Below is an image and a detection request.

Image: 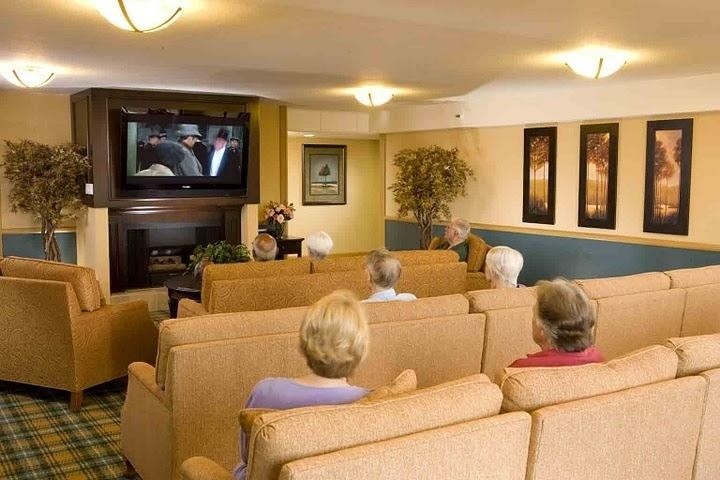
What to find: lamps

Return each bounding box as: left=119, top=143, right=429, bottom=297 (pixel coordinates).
left=87, top=0, right=196, bottom=39
left=556, top=43, right=633, bottom=81
left=350, top=84, right=398, bottom=110
left=0, top=57, right=62, bottom=94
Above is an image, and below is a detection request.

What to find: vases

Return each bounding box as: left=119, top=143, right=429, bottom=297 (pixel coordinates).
left=264, top=223, right=286, bottom=239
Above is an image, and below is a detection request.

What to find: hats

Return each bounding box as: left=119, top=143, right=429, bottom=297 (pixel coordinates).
left=148, top=131, right=161, bottom=138
left=216, top=128, right=230, bottom=141
left=149, top=142, right=185, bottom=166
left=174, top=124, right=202, bottom=137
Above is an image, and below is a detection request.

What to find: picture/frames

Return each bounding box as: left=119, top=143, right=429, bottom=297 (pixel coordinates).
left=521, top=122, right=558, bottom=227
left=299, top=141, right=349, bottom=209
left=574, top=123, right=620, bottom=229
left=642, top=114, right=695, bottom=237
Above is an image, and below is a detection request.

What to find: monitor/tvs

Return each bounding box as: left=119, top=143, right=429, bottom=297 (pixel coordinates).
left=120, top=113, right=249, bottom=189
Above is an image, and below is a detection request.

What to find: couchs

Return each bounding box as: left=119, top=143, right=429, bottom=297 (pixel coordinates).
left=665, top=330, right=719, bottom=478
left=116, top=292, right=490, bottom=477
left=661, top=263, right=719, bottom=336
left=171, top=244, right=472, bottom=315
left=175, top=365, right=532, bottom=480
left=0, top=246, right=164, bottom=413
left=461, top=269, right=689, bottom=379
left=488, top=343, right=711, bottom=480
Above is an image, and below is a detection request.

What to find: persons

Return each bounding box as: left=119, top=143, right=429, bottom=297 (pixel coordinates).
left=485, top=246, right=529, bottom=289
left=356, top=248, right=417, bottom=303
left=251, top=235, right=279, bottom=260
left=436, top=218, right=471, bottom=262
left=234, top=288, right=372, bottom=478
left=306, top=229, right=333, bottom=257
left=135, top=125, right=243, bottom=177
left=509, top=275, right=607, bottom=367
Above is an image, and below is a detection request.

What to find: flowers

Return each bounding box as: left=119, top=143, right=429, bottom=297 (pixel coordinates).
left=261, top=197, right=300, bottom=225
